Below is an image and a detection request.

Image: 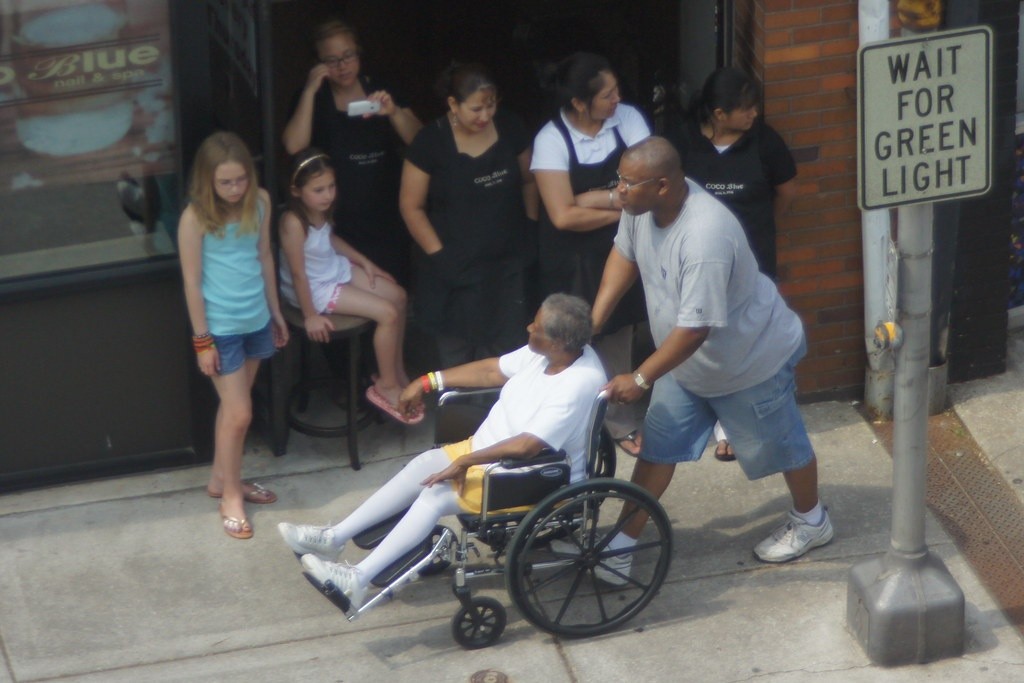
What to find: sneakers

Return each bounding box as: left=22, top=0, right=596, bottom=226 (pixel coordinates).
left=278, top=522, right=345, bottom=563
left=301, top=553, right=367, bottom=611
left=549, top=532, right=632, bottom=587
left=753, top=503, right=833, bottom=564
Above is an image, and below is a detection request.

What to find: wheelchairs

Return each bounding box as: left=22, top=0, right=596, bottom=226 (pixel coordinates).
left=294, top=384, right=674, bottom=652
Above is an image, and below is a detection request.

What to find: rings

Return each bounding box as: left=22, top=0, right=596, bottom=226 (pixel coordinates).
left=617, top=400, right=624, bottom=405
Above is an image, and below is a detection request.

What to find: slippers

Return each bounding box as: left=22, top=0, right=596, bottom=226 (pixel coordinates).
left=219, top=502, right=253, bottom=539
left=366, top=386, right=424, bottom=424
left=207, top=483, right=277, bottom=503
left=371, top=373, right=426, bottom=412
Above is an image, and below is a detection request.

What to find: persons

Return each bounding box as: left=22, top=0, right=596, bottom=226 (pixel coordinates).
left=665, top=68, right=799, bottom=460
left=524, top=50, right=652, bottom=457
left=276, top=147, right=426, bottom=426
left=278, top=291, right=608, bottom=609
left=588, top=137, right=835, bottom=587
left=396, top=67, right=538, bottom=391
left=176, top=131, right=290, bottom=540
left=282, top=20, right=439, bottom=371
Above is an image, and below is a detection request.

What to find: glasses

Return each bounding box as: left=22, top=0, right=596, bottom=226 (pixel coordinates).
left=316, top=47, right=360, bottom=68
left=616, top=170, right=661, bottom=193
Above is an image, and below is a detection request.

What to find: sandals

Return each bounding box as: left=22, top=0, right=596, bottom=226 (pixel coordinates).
left=613, top=429, right=642, bottom=456
left=715, top=438, right=735, bottom=461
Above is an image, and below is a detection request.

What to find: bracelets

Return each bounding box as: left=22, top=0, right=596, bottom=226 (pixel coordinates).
left=427, top=372, right=437, bottom=390
left=633, top=369, right=650, bottom=390
left=420, top=375, right=431, bottom=394
left=191, top=331, right=216, bottom=355
left=435, top=370, right=443, bottom=391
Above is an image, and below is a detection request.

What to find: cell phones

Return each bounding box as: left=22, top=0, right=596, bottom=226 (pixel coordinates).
left=348, top=99, right=380, bottom=116
